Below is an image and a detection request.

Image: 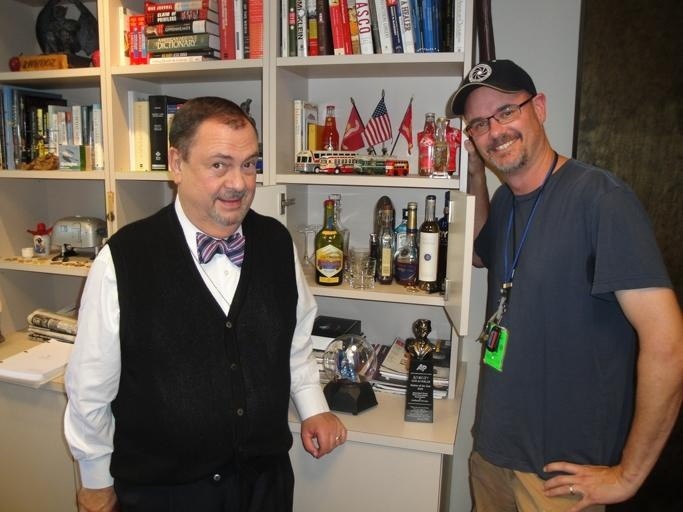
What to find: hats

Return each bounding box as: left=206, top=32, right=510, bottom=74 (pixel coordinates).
left=451, top=59, right=536, bottom=115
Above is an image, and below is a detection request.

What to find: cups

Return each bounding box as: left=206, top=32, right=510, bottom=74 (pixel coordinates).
left=22, top=247, right=33, bottom=259
left=345, top=247, right=376, bottom=290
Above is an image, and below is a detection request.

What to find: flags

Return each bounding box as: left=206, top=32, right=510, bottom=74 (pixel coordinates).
left=398, top=103, right=413, bottom=155
left=341, top=106, right=365, bottom=152
left=363, top=96, right=394, bottom=148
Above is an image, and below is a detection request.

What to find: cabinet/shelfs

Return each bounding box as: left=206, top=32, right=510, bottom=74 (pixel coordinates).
left=0, top=0, right=472, bottom=512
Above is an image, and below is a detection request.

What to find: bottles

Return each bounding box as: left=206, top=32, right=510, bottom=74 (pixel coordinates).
left=436, top=191, right=449, bottom=292
left=416, top=194, right=441, bottom=294
left=394, top=201, right=420, bottom=288
left=313, top=193, right=351, bottom=287
left=321, top=105, right=339, bottom=150
left=367, top=233, right=376, bottom=278
left=393, top=208, right=407, bottom=235
left=375, top=203, right=397, bottom=285
left=32, top=222, right=51, bottom=257
left=417, top=112, right=461, bottom=178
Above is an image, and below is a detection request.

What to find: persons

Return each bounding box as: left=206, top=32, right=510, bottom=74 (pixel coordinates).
left=450, top=56, right=683, bottom=512
left=63, top=95, right=348, bottom=512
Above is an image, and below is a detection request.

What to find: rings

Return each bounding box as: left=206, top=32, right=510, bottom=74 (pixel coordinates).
left=569, top=484, right=575, bottom=495
left=336, top=435, right=343, bottom=441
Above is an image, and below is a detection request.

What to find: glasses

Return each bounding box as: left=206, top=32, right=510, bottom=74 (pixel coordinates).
left=466, top=97, right=533, bottom=137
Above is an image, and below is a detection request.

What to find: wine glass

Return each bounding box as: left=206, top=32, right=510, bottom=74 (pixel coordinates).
left=296, top=222, right=314, bottom=280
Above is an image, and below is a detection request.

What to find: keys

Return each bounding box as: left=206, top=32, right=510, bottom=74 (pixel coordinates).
left=475, top=320, right=501, bottom=353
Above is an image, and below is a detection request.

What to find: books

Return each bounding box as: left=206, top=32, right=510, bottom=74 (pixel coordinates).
left=310, top=314, right=451, bottom=400
left=8, top=51, right=91, bottom=72
left=0, top=85, right=189, bottom=172
left=125, top=2, right=264, bottom=66
left=293, top=99, right=320, bottom=172
left=279, top=2, right=455, bottom=57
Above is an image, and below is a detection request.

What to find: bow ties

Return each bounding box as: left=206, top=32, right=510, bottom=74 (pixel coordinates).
left=196, top=232, right=245, bottom=267
